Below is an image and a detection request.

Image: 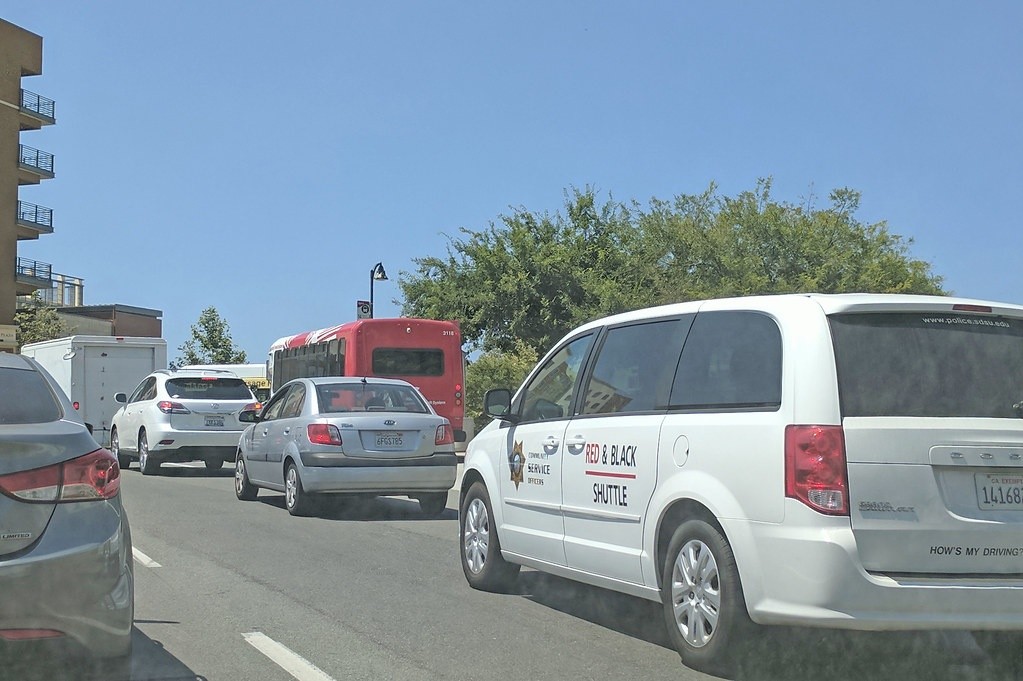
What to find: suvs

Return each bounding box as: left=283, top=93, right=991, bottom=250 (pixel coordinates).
left=109, top=368, right=266, bottom=477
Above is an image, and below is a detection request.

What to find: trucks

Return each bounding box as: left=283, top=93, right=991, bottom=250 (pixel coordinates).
left=20, top=335, right=168, bottom=450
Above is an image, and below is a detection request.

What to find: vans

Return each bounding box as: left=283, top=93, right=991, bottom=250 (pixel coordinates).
left=458, top=293, right=1023, bottom=674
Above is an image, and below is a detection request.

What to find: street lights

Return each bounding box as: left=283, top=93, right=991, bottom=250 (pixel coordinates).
left=371, top=261, right=388, bottom=320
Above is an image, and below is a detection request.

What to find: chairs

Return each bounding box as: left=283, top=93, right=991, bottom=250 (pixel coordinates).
left=364, top=398, right=385, bottom=410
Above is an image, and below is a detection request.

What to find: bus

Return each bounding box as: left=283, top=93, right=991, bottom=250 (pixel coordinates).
left=266, top=317, right=464, bottom=432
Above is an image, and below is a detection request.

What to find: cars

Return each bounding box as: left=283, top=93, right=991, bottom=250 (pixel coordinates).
left=0, top=353, right=134, bottom=681
left=233, top=376, right=458, bottom=518
